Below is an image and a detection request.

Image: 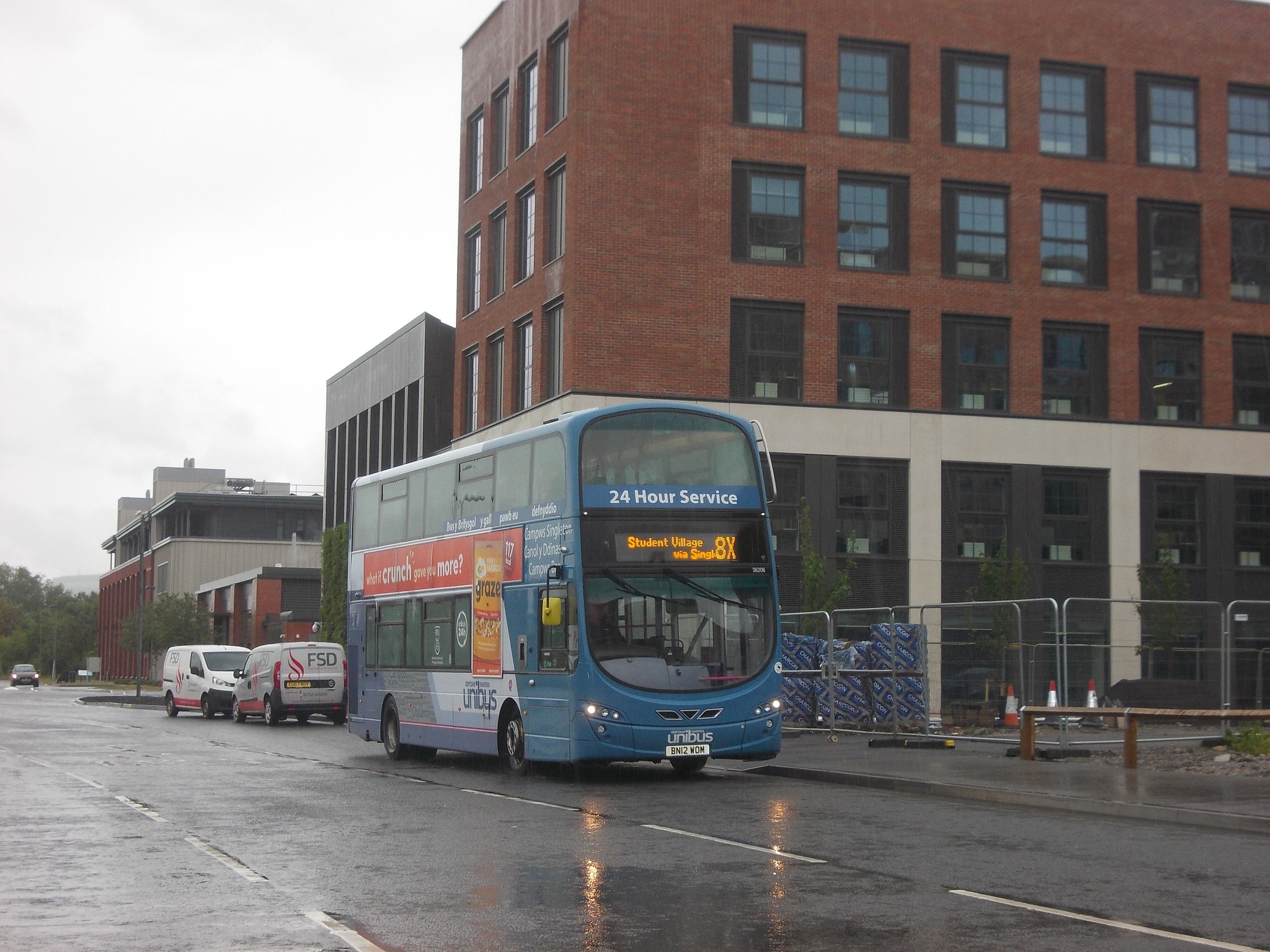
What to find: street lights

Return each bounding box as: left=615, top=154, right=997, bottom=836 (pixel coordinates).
left=49, top=605, right=57, bottom=678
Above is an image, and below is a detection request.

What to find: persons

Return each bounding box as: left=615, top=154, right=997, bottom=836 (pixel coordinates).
left=586, top=602, right=627, bottom=652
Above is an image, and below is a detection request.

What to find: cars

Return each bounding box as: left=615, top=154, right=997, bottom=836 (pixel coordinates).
left=10, top=664, right=41, bottom=687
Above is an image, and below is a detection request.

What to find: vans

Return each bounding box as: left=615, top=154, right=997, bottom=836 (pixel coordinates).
left=162, top=645, right=252, bottom=719
left=231, top=641, right=348, bottom=727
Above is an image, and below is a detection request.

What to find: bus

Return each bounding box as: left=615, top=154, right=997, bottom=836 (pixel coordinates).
left=347, top=402, right=784, bottom=778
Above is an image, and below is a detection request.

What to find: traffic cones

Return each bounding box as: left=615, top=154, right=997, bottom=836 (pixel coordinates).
left=1036, top=680, right=1068, bottom=726
left=1075, top=679, right=1105, bottom=727
left=997, top=684, right=1020, bottom=730
